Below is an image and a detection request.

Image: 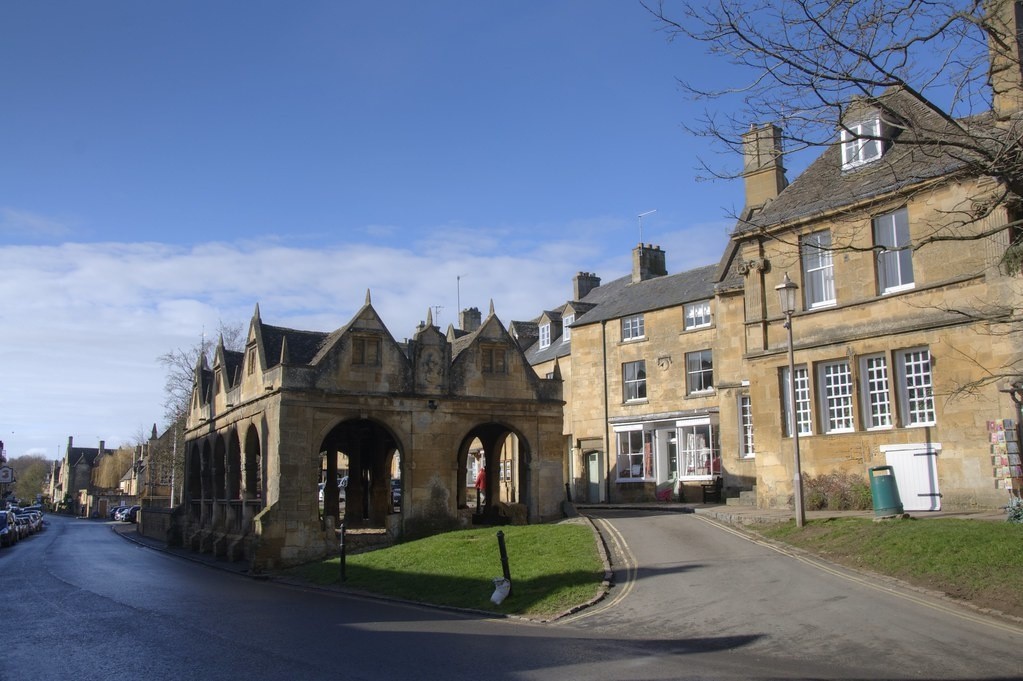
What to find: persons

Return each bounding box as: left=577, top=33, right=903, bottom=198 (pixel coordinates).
left=7, top=508, right=15, bottom=526
left=475, top=467, right=486, bottom=505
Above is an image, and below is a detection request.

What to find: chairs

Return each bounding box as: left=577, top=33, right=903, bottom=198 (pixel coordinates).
left=701, top=476, right=720, bottom=503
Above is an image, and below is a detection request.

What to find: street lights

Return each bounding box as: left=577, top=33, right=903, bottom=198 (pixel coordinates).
left=775, top=272, right=805, bottom=527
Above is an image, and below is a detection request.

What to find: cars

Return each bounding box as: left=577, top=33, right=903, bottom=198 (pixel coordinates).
left=110, top=505, right=141, bottom=524
left=0, top=505, right=44, bottom=548
left=390, top=480, right=402, bottom=506
left=317, top=476, right=349, bottom=509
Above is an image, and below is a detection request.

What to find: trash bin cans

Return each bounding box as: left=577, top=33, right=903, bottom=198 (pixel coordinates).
left=868, top=465, right=904, bottom=520
